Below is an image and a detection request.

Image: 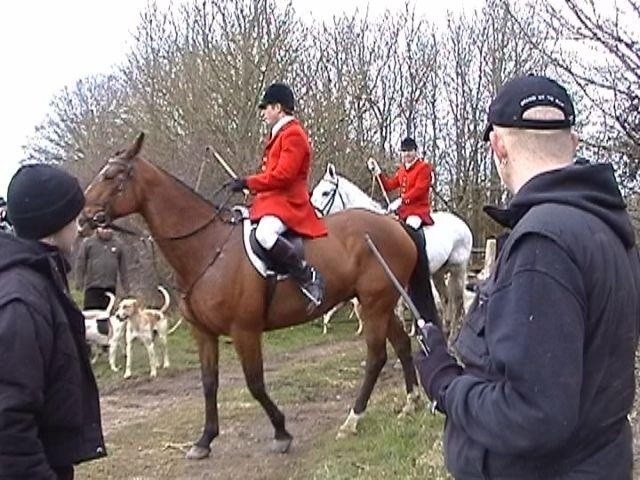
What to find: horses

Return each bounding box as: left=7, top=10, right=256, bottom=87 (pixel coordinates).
left=74, top=130, right=443, bottom=461
left=310, top=162, right=474, bottom=370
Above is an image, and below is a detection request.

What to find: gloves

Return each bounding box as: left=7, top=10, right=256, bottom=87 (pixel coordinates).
left=389, top=197, right=402, bottom=211
left=367, top=157, right=381, bottom=177
left=230, top=178, right=246, bottom=192
left=407, top=325, right=466, bottom=414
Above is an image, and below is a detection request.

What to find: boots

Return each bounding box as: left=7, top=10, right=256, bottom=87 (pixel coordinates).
left=268, top=237, right=323, bottom=314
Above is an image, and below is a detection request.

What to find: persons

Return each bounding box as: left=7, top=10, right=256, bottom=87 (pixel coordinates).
left=366, top=139, right=434, bottom=231
left=0, top=164, right=107, bottom=480
left=221, top=84, right=328, bottom=316
left=411, top=75, right=640, bottom=480
left=75, top=229, right=130, bottom=310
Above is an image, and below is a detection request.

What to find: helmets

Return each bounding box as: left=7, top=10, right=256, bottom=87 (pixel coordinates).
left=399, top=137, right=418, bottom=151
left=259, top=81, right=295, bottom=116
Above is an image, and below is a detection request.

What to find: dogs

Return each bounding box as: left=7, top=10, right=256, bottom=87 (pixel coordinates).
left=81, top=292, right=128, bottom=372
left=114, top=285, right=171, bottom=379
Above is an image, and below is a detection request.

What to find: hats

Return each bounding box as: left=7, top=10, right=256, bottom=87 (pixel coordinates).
left=484, top=72, right=575, bottom=143
left=7, top=165, right=85, bottom=240
